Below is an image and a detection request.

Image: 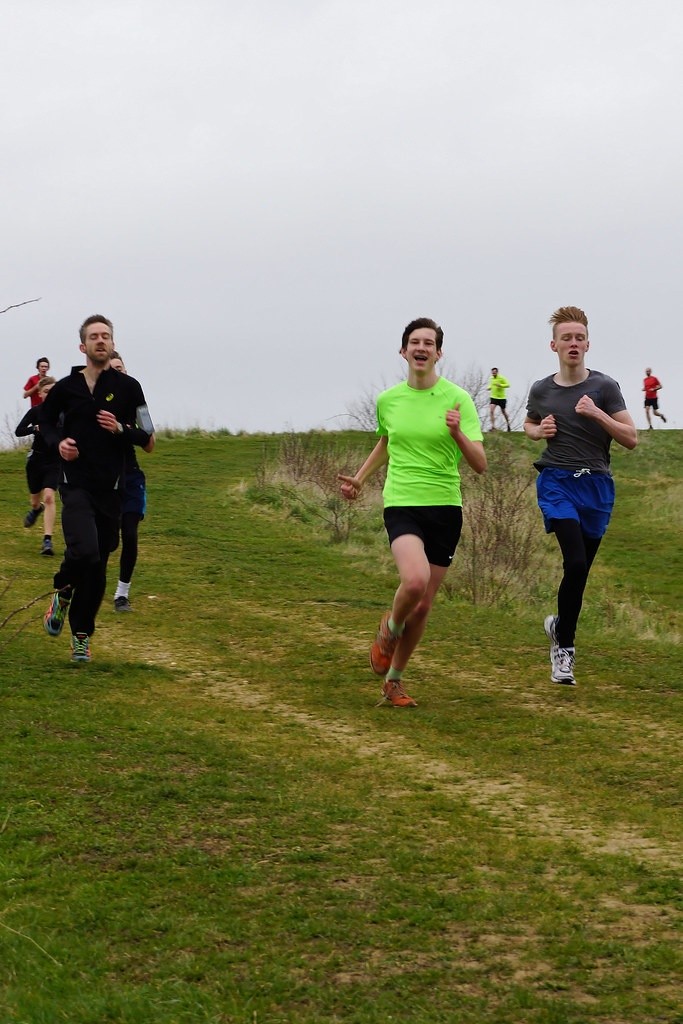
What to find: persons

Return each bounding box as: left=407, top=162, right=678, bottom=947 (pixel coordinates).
left=339, top=317, right=487, bottom=708
left=15, top=316, right=155, bottom=662
left=486, top=368, right=511, bottom=433
left=523, top=307, right=638, bottom=683
left=642, top=368, right=667, bottom=429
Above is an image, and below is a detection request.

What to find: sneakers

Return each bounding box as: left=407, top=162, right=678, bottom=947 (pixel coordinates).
left=544, top=615, right=562, bottom=645
left=370, top=612, right=399, bottom=676
left=549, top=644, right=576, bottom=685
left=24, top=502, right=44, bottom=527
left=39, top=539, right=55, bottom=557
left=114, top=596, right=130, bottom=611
left=381, top=678, right=418, bottom=708
left=70, top=632, right=91, bottom=663
left=44, top=587, right=73, bottom=636
